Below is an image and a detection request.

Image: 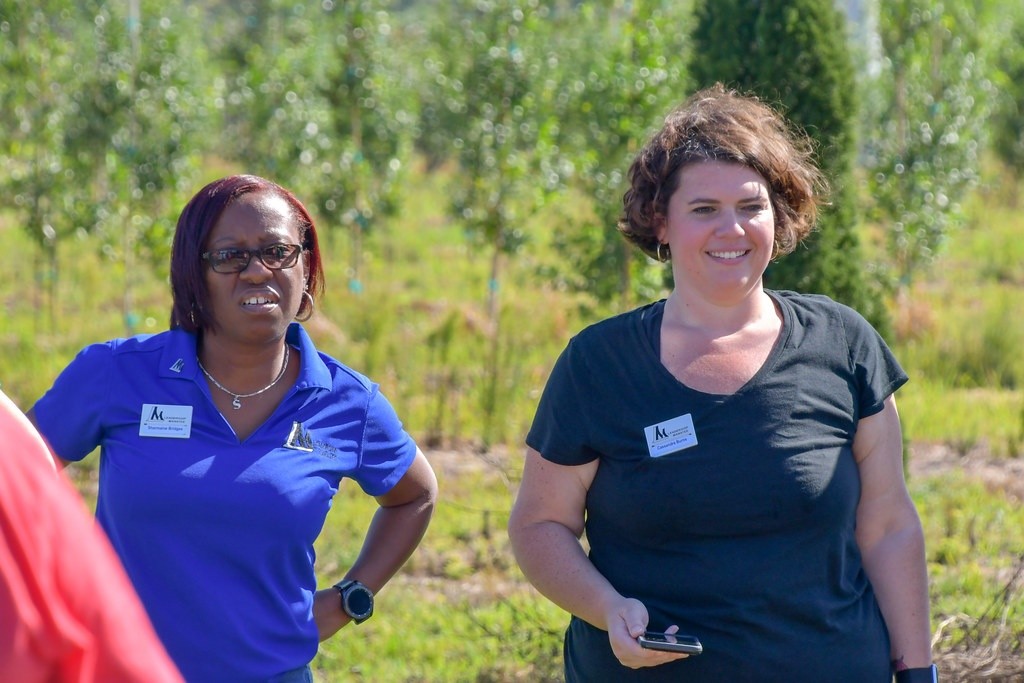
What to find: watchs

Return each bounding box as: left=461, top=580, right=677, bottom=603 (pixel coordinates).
left=896, top=663, right=938, bottom=683
left=334, top=580, right=374, bottom=625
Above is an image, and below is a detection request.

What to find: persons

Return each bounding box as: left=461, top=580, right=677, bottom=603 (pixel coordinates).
left=508, top=86, right=931, bottom=683
left=26, top=175, right=439, bottom=683
left=0, top=393, right=182, bottom=683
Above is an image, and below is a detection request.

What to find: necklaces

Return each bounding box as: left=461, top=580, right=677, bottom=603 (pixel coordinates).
left=197, top=342, right=290, bottom=408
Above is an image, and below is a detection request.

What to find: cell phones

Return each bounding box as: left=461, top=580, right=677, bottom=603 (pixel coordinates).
left=636, top=631, right=703, bottom=655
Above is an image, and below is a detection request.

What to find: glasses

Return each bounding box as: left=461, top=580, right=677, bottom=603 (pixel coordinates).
left=201, top=243, right=303, bottom=274
